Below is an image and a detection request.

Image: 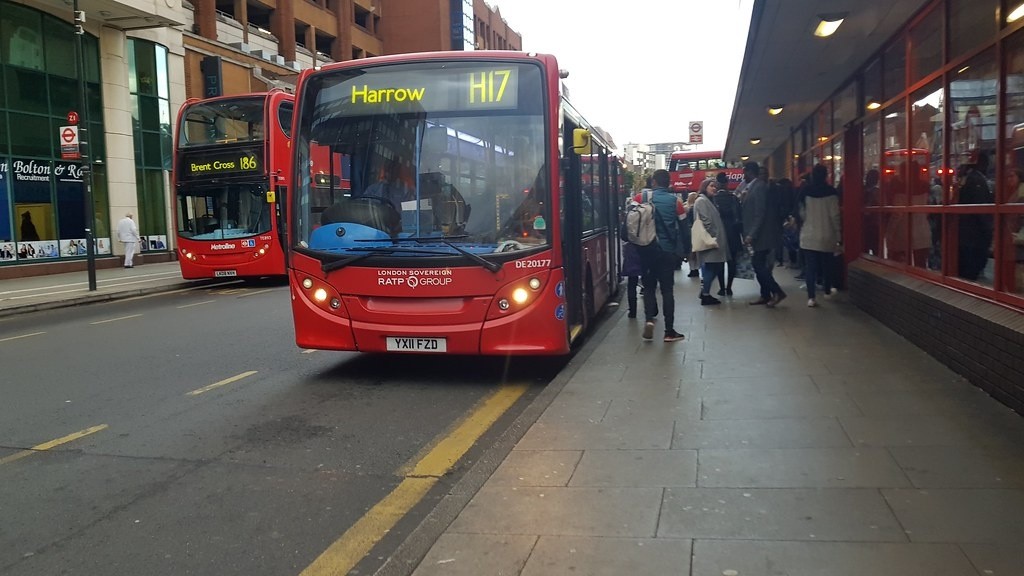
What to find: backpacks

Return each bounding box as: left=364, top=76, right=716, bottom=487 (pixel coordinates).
left=626, top=189, right=656, bottom=246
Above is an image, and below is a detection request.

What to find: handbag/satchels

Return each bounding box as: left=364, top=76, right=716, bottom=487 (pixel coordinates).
left=691, top=197, right=718, bottom=252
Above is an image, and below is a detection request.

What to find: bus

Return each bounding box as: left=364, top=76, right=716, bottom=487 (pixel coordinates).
left=172, top=87, right=416, bottom=283
left=287, top=50, right=626, bottom=355
left=880, top=149, right=933, bottom=195
left=665, top=150, right=748, bottom=205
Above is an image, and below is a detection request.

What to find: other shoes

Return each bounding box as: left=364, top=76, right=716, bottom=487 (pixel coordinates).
left=815, top=284, right=824, bottom=289
left=768, top=291, right=787, bottom=306
left=688, top=269, right=699, bottom=277
left=640, top=288, right=645, bottom=295
left=808, top=298, right=816, bottom=306
left=794, top=272, right=807, bottom=280
left=699, top=293, right=721, bottom=305
left=675, top=264, right=681, bottom=270
left=726, top=289, right=733, bottom=295
left=749, top=294, right=771, bottom=304
left=800, top=283, right=807, bottom=289
left=824, top=287, right=837, bottom=300
left=628, top=311, right=637, bottom=318
left=717, top=289, right=725, bottom=295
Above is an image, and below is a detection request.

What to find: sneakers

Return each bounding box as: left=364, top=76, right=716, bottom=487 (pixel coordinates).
left=664, top=328, right=684, bottom=341
left=642, top=317, right=656, bottom=340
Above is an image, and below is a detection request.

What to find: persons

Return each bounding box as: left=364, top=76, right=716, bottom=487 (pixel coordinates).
left=361, top=158, right=416, bottom=215
left=140, top=237, right=147, bottom=249
left=21, top=211, right=40, bottom=241
left=156, top=236, right=165, bottom=249
left=1, top=244, right=13, bottom=260
left=39, top=245, right=57, bottom=257
left=621, top=160, right=843, bottom=342
left=67, top=240, right=87, bottom=255
left=864, top=166, right=1024, bottom=293
left=18, top=244, right=35, bottom=258
left=116, top=213, right=143, bottom=268
left=95, top=212, right=106, bottom=237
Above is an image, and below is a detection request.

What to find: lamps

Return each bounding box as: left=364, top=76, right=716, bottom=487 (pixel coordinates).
left=808, top=11, right=849, bottom=37
left=766, top=102, right=787, bottom=115
